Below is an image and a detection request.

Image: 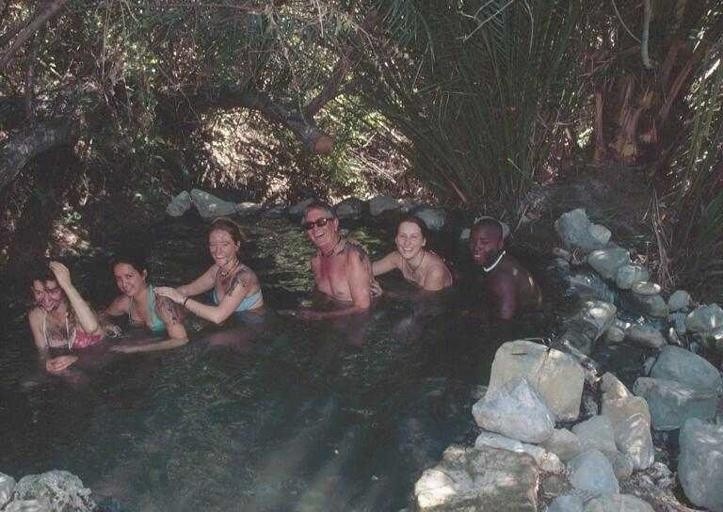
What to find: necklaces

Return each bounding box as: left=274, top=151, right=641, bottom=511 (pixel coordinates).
left=404, top=248, right=427, bottom=280
left=218, top=263, right=237, bottom=279
left=480, top=247, right=509, bottom=273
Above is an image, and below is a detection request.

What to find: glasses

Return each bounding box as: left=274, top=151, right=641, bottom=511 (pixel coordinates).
left=305, top=217, right=333, bottom=229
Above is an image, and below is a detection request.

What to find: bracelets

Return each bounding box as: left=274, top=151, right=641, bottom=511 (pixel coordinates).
left=183, top=295, right=192, bottom=307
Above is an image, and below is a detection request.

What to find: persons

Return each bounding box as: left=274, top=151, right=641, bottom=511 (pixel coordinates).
left=345, top=215, right=457, bottom=338
left=275, top=202, right=375, bottom=348
left=97, top=258, right=193, bottom=355
left=27, top=260, right=108, bottom=387
left=467, top=215, right=543, bottom=342
left=147, top=215, right=268, bottom=350
